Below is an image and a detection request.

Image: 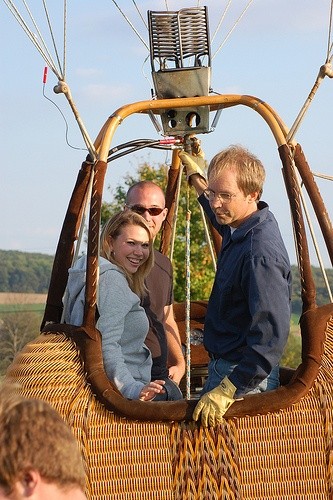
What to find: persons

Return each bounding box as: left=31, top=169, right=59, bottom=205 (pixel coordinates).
left=176, top=145, right=293, bottom=430
left=102, top=182, right=186, bottom=386
left=60, top=207, right=184, bottom=403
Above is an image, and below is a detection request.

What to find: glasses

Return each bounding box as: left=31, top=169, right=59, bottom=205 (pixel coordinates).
left=126, top=205, right=164, bottom=216
left=203, top=189, right=242, bottom=204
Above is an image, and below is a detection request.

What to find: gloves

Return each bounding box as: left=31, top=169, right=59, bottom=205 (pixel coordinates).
left=191, top=375, right=246, bottom=427
left=179, top=153, right=207, bottom=187
